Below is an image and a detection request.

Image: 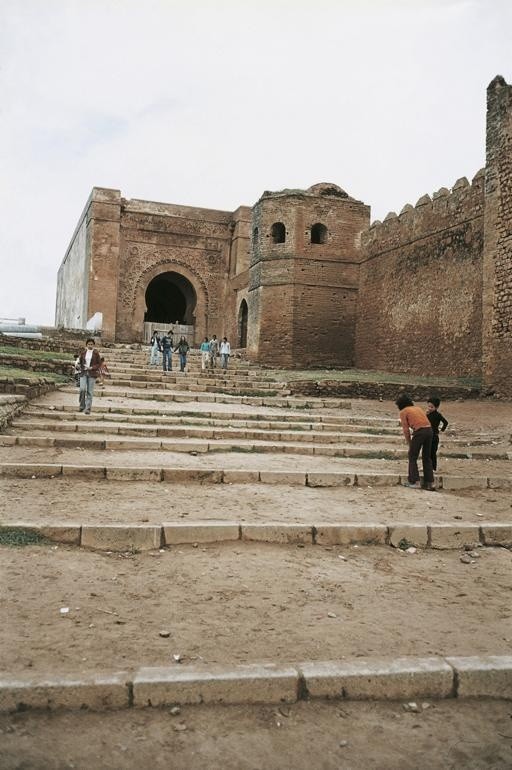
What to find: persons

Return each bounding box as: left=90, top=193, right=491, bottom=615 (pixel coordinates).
left=200, top=336, right=212, bottom=370
left=98, top=357, right=109, bottom=378
left=394, top=394, right=438, bottom=492
left=218, top=336, right=232, bottom=369
left=174, top=335, right=191, bottom=372
left=160, top=330, right=175, bottom=372
left=75, top=337, right=101, bottom=415
left=209, top=334, right=220, bottom=368
left=422, top=396, right=448, bottom=472
left=150, top=330, right=162, bottom=365
left=73, top=354, right=82, bottom=387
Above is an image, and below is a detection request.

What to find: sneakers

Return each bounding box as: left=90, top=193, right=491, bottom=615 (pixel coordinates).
left=79, top=404, right=91, bottom=414
left=404, top=481, right=436, bottom=491
left=420, top=467, right=437, bottom=472
